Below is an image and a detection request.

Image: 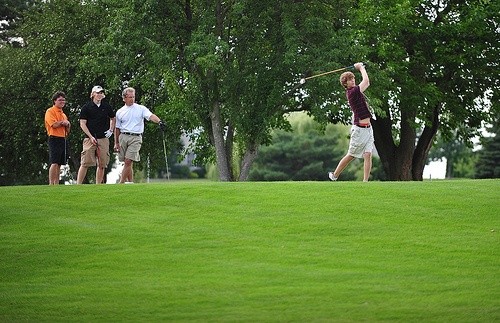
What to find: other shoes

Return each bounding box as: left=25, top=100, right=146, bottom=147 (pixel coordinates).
left=328, top=172, right=337, bottom=181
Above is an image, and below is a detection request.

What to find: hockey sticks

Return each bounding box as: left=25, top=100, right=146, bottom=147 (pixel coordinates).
left=300, top=65, right=354, bottom=84
left=64, top=127, right=67, bottom=171
left=162, top=129, right=169, bottom=182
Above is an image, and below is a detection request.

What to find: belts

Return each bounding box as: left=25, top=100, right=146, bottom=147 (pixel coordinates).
left=356, top=124, right=371, bottom=129
left=123, top=131, right=141, bottom=137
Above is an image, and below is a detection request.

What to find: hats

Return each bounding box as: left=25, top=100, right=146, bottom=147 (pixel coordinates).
left=92, top=86, right=105, bottom=93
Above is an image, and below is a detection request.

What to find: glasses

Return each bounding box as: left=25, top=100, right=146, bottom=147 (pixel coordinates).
left=57, top=99, right=66, bottom=102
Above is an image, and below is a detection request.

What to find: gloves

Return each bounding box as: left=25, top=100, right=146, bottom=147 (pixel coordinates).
left=104, top=128, right=113, bottom=138
left=159, top=120, right=166, bottom=131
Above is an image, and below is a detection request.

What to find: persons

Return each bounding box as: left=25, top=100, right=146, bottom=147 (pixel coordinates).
left=76, top=86, right=113, bottom=184
left=116, top=88, right=167, bottom=184
left=45, top=91, right=71, bottom=185
left=328, top=61, right=375, bottom=181
